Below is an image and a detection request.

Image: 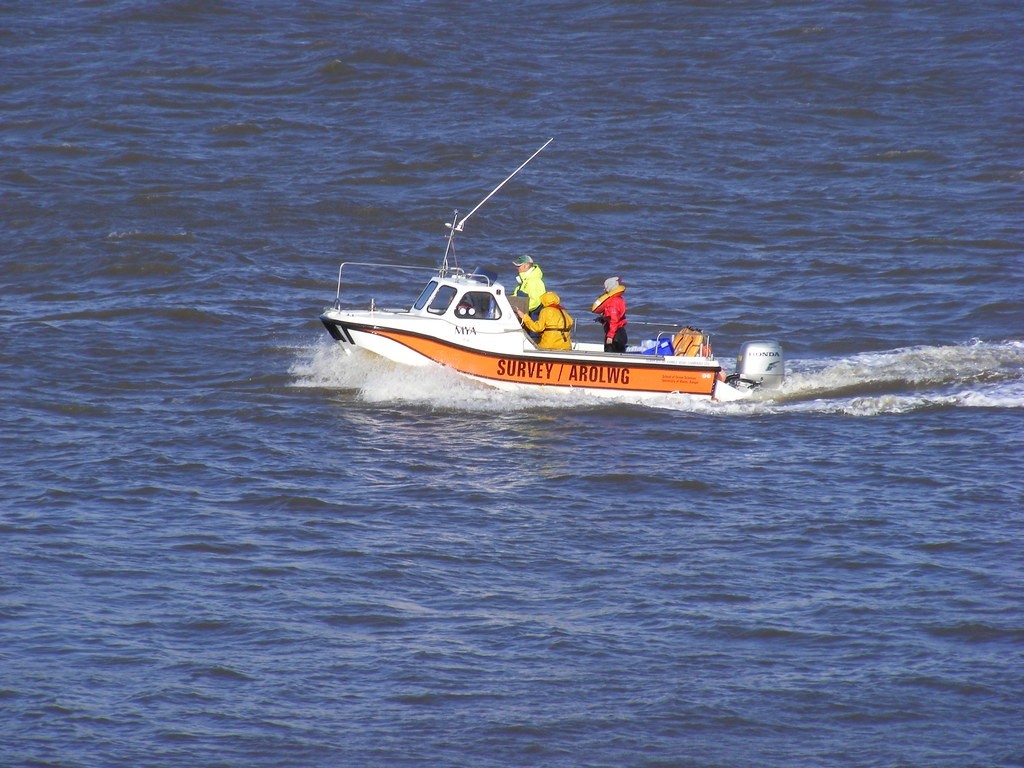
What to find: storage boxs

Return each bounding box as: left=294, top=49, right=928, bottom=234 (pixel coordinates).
left=625, top=337, right=673, bottom=356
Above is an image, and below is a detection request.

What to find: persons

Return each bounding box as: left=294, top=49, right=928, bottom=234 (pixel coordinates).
left=509, top=291, right=574, bottom=351
left=510, top=255, right=549, bottom=338
left=601, top=277, right=631, bottom=354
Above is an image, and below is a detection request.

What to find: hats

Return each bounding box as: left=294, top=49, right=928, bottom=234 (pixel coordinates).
left=512, top=255, right=533, bottom=266
left=604, top=277, right=622, bottom=292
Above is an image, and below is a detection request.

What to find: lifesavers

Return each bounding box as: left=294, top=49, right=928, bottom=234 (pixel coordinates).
left=591, top=285, right=626, bottom=315
left=672, top=328, right=704, bottom=357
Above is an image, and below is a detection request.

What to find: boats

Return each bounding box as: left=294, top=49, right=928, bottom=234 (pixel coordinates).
left=318, top=133, right=788, bottom=401
